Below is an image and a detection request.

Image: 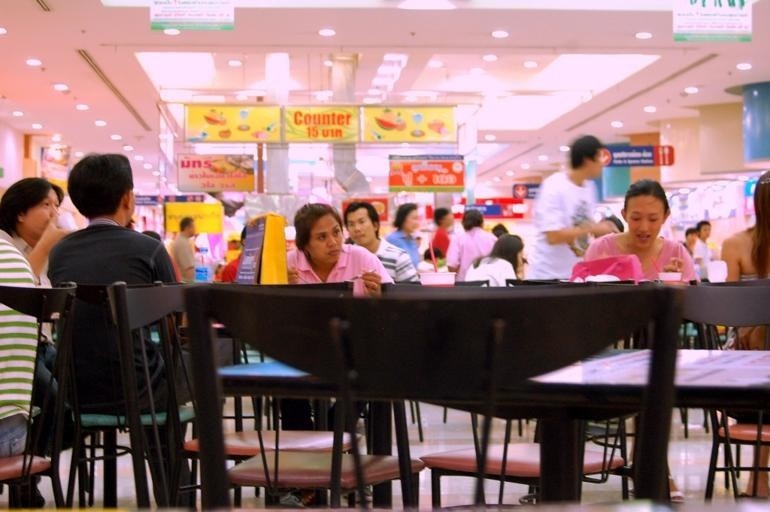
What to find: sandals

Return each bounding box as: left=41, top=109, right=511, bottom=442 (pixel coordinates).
left=668, top=474, right=683, bottom=503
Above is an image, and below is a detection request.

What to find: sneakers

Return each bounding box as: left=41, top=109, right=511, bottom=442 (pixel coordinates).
left=281, top=493, right=304, bottom=506
left=349, top=486, right=372, bottom=502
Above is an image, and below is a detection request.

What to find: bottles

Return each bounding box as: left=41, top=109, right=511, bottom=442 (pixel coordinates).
left=194, top=232, right=208, bottom=281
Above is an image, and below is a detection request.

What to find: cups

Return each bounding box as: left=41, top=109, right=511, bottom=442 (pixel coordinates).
left=417, top=272, right=458, bottom=288
left=657, top=272, right=683, bottom=284
left=55, top=211, right=78, bottom=233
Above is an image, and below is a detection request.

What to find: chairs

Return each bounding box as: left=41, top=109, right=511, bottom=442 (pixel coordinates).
left=660, top=275, right=724, bottom=439
left=691, top=276, right=769, bottom=507
left=414, top=408, right=629, bottom=508
left=116, top=281, right=360, bottom=512
left=0, top=281, right=77, bottom=509
left=696, top=383, right=770, bottom=498
left=215, top=431, right=425, bottom=512
left=65, top=283, right=198, bottom=511
left=183, top=398, right=359, bottom=458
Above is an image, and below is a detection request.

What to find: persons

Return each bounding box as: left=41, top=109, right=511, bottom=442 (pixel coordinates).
left=170, top=214, right=198, bottom=283
left=45, top=153, right=225, bottom=512
left=490, top=224, right=509, bottom=238
left=210, top=224, right=246, bottom=282
left=0, top=177, right=71, bottom=287
left=429, top=204, right=461, bottom=270
left=384, top=201, right=424, bottom=278
left=721, top=170, right=770, bottom=350
left=692, top=220, right=712, bottom=280
left=677, top=227, right=698, bottom=256
left=341, top=200, right=420, bottom=287
left=464, top=233, right=524, bottom=287
left=444, top=209, right=495, bottom=284
left=283, top=203, right=395, bottom=298
left=416, top=244, right=444, bottom=277
left=524, top=133, right=619, bottom=280
left=0, top=237, right=37, bottom=458
left=584, top=179, right=699, bottom=285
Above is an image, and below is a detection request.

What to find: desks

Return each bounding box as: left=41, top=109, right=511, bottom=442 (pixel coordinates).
left=507, top=334, right=770, bottom=512
left=184, top=269, right=679, bottom=512
